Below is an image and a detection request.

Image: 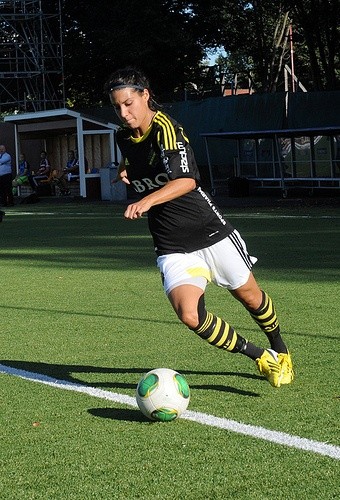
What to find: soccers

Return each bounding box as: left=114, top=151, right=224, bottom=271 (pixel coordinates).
left=136, top=368, right=191, bottom=422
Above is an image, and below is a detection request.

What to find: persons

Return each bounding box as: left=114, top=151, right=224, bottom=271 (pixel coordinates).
left=0, top=145, right=14, bottom=207
left=27, top=150, right=50, bottom=194
left=11, top=154, right=30, bottom=196
left=106, top=69, right=294, bottom=387
left=56, top=148, right=88, bottom=197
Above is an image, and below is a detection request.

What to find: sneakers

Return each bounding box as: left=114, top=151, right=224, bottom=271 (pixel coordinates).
left=257, top=348, right=284, bottom=388
left=279, top=350, right=294, bottom=384
